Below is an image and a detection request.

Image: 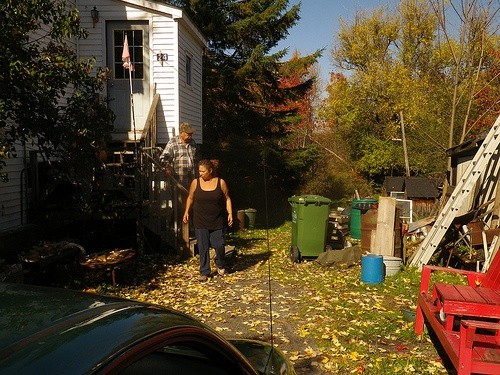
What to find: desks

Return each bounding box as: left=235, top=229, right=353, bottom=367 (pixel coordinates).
left=79, top=248, right=135, bottom=287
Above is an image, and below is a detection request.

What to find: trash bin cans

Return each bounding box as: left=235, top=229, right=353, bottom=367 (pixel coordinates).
left=233, top=210, right=245, bottom=229
left=349, top=198, right=377, bottom=239
left=287, top=194, right=332, bottom=262
left=245, top=208, right=257, bottom=230
left=354, top=204, right=403, bottom=258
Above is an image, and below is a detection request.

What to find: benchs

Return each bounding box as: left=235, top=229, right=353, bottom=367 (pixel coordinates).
left=414, top=249, right=500, bottom=375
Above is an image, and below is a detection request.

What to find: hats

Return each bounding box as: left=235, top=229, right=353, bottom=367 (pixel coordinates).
left=180, top=122, right=196, bottom=133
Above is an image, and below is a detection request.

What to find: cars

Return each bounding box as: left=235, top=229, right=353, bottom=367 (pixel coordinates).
left=0, top=280, right=297, bottom=375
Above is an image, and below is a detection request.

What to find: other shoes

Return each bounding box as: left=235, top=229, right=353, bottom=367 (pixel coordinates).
left=198, top=275, right=208, bottom=282
left=216, top=264, right=225, bottom=275
left=170, top=214, right=175, bottom=228
left=188, top=229, right=195, bottom=240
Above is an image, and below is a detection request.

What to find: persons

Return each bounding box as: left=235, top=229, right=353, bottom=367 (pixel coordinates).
left=183, top=159, right=233, bottom=280
left=159, top=122, right=199, bottom=230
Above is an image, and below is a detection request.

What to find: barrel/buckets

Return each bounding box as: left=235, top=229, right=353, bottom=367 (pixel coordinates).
left=361, top=253, right=384, bottom=284
left=350, top=199, right=378, bottom=240
left=382, top=256, right=402, bottom=276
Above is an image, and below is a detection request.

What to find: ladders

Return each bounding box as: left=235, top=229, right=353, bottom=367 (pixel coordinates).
left=408, top=114, right=500, bottom=272
